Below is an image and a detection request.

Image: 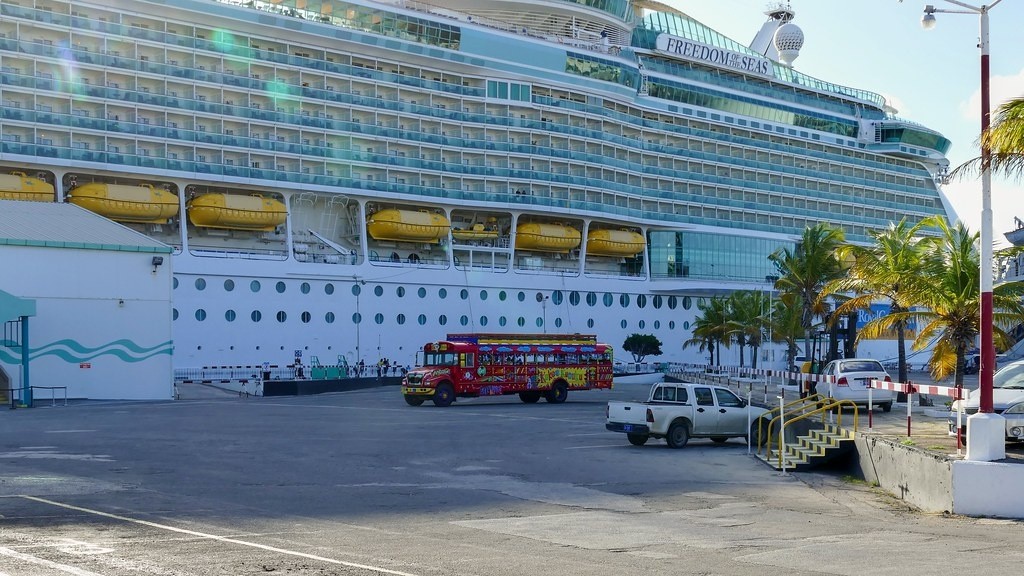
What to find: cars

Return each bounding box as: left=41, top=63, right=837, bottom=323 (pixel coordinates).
left=948, top=360, right=1024, bottom=447
left=814, top=358, right=894, bottom=414
left=794, top=356, right=806, bottom=373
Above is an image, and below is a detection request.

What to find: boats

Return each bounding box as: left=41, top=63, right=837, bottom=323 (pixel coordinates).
left=367, top=209, right=449, bottom=243
left=586, top=229, right=645, bottom=258
left=453, top=217, right=499, bottom=241
left=1, top=171, right=54, bottom=202
left=514, top=223, right=581, bottom=254
left=186, top=192, right=287, bottom=232
left=68, top=182, right=179, bottom=225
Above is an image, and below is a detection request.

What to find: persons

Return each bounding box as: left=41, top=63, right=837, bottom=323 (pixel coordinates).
left=393, top=360, right=397, bottom=377
left=522, top=190, right=526, bottom=194
left=354, top=359, right=364, bottom=376
left=516, top=189, right=521, bottom=194
left=377, top=358, right=389, bottom=375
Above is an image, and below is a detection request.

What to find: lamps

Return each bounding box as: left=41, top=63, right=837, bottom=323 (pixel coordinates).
left=152, top=256, right=163, bottom=268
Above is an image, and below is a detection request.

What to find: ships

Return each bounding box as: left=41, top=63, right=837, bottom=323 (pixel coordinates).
left=0, top=0, right=981, bottom=398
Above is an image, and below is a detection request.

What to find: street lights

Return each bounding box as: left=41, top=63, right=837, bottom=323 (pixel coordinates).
left=353, top=274, right=366, bottom=364
left=542, top=296, right=546, bottom=332
left=898, top=0, right=1005, bottom=461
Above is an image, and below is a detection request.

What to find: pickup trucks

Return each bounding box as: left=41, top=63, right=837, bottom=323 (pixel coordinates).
left=606, top=383, right=774, bottom=449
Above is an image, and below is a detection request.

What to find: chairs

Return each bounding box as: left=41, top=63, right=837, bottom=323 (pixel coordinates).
left=702, top=392, right=713, bottom=404
left=660, top=388, right=670, bottom=399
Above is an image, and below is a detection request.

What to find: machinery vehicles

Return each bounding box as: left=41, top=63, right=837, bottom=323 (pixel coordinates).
left=799, top=311, right=857, bottom=401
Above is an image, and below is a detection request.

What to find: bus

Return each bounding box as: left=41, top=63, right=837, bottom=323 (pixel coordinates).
left=402, top=333, right=614, bottom=407
